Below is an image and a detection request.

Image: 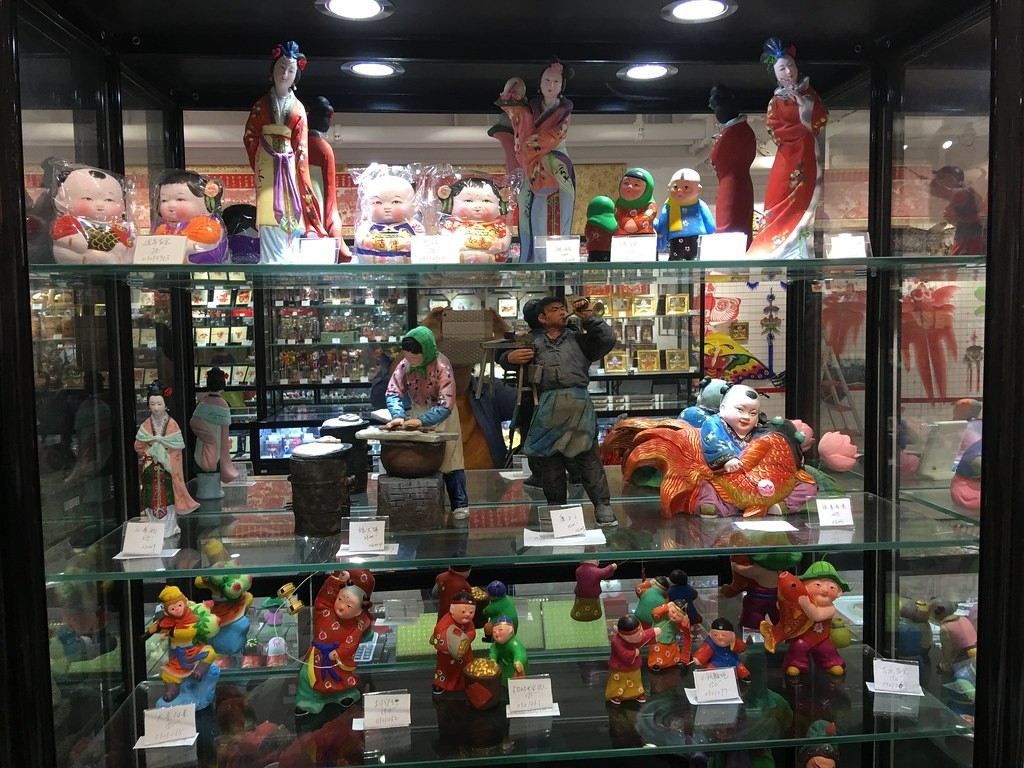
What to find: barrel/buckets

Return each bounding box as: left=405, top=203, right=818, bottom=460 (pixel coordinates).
left=288, top=456, right=352, bottom=538
left=320, top=420, right=373, bottom=494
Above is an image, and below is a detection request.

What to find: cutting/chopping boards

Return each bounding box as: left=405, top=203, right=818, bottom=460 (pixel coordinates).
left=355, top=428, right=459, bottom=443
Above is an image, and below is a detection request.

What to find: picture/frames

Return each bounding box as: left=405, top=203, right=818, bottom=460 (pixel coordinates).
left=604, top=350, right=627, bottom=374
left=665, top=293, right=689, bottom=316
left=730, top=322, right=750, bottom=340
left=637, top=349, right=661, bottom=372
left=497, top=297, right=518, bottom=320
left=665, top=349, right=689, bottom=372
left=589, top=294, right=613, bottom=317
left=631, top=295, right=655, bottom=317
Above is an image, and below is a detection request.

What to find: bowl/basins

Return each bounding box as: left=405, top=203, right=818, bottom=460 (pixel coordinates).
left=379, top=439, right=446, bottom=478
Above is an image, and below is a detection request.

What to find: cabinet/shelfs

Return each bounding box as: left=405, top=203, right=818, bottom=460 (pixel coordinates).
left=0, top=0, right=1021, bottom=767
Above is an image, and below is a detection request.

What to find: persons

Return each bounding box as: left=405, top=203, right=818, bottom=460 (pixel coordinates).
left=49, top=34, right=987, bottom=264
left=136, top=546, right=976, bottom=721
left=114, top=295, right=982, bottom=533
left=796, top=721, right=844, bottom=768
